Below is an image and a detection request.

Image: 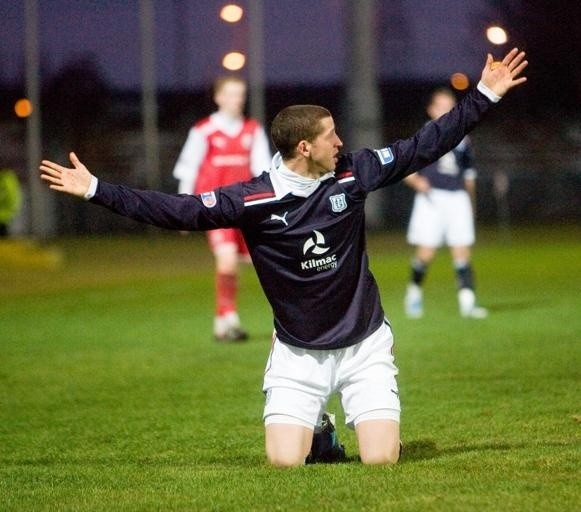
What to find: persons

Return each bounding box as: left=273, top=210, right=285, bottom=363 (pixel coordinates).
left=168, top=73, right=276, bottom=343
left=0, top=159, right=26, bottom=238
left=393, top=86, right=492, bottom=322
left=38, top=46, right=531, bottom=468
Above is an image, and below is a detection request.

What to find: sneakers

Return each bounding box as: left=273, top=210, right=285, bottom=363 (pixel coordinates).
left=403, top=302, right=491, bottom=318
left=213, top=327, right=249, bottom=342
left=308, top=411, right=348, bottom=463
